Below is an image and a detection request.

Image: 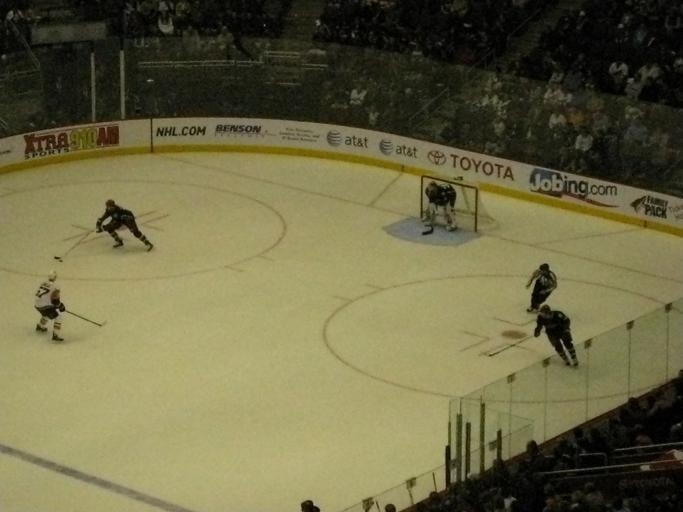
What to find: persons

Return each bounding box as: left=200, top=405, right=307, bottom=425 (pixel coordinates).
left=1, top=0, right=298, bottom=138
left=419, top=179, right=458, bottom=232
left=298, top=369, right=682, bottom=512
left=303, top=0, right=682, bottom=200
left=534, top=304, right=578, bottom=367
left=94, top=200, right=153, bottom=252
left=525, top=262, right=557, bottom=312
left=33, top=270, right=65, bottom=341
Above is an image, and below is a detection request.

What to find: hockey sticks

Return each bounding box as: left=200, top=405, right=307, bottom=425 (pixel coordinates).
left=54, top=227, right=96, bottom=260
left=481, top=336, right=534, bottom=357
left=422, top=210, right=435, bottom=234
left=65, top=310, right=106, bottom=327
